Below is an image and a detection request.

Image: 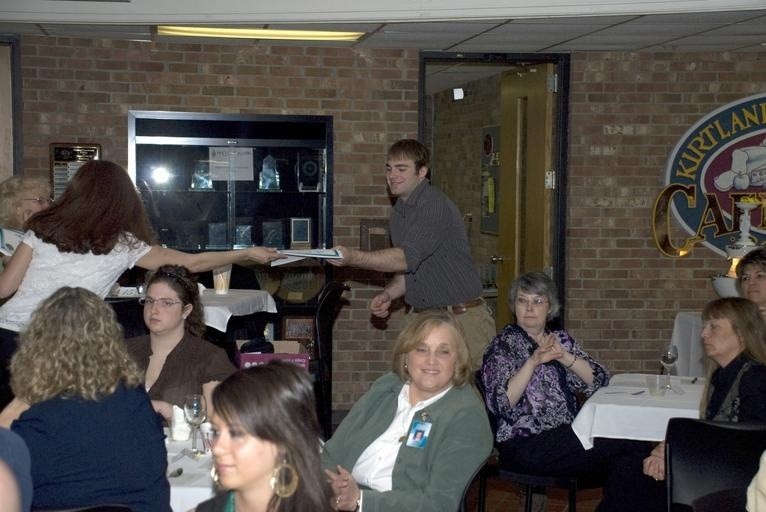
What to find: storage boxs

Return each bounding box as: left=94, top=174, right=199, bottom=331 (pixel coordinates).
left=231, top=338, right=310, bottom=374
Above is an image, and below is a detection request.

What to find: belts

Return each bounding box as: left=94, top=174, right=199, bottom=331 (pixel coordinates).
left=413, top=299, right=483, bottom=314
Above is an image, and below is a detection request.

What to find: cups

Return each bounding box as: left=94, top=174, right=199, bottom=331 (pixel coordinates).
left=211, top=261, right=234, bottom=295
left=166, top=415, right=194, bottom=443
left=646, top=374, right=671, bottom=398
left=198, top=421, right=214, bottom=455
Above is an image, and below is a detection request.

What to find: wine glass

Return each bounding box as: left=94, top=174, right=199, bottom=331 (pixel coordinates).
left=657, top=344, right=680, bottom=395
left=181, top=392, right=209, bottom=459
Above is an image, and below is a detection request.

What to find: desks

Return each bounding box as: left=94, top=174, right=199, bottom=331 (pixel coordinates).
left=668, top=310, right=705, bottom=375
left=108, top=288, right=277, bottom=343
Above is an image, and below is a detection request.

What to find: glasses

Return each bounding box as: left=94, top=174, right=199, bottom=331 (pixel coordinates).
left=518, top=297, right=548, bottom=306
left=23, top=196, right=53, bottom=205
left=138, top=297, right=179, bottom=307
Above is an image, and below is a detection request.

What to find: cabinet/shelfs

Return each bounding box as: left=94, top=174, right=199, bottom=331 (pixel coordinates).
left=126, top=108, right=335, bottom=436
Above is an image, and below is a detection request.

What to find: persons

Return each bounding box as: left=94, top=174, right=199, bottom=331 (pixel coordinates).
left=321, top=137, right=499, bottom=377
left=0, top=426, right=33, bottom=511
left=126, top=264, right=238, bottom=427
left=736, top=248, right=766, bottom=319
left=196, top=362, right=334, bottom=511
left=1, top=175, right=52, bottom=267
left=415, top=429, right=424, bottom=440
left=316, top=308, right=494, bottom=512
left=0, top=160, right=288, bottom=414
left=595, top=295, right=766, bottom=512
left=10, top=286, right=173, bottom=511
left=481, top=271, right=661, bottom=511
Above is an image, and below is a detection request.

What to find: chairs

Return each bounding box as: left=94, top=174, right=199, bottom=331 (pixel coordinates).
left=470, top=377, right=601, bottom=511
left=664, top=415, right=766, bottom=512
left=272, top=281, right=351, bottom=442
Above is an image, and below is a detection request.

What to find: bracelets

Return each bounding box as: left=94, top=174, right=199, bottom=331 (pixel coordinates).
left=353, top=497, right=361, bottom=512
left=566, top=353, right=579, bottom=368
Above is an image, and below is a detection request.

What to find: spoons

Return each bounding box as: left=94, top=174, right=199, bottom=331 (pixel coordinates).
left=604, top=390, right=647, bottom=396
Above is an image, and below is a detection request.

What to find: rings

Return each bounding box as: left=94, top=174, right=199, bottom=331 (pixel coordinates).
left=335, top=494, right=342, bottom=503
